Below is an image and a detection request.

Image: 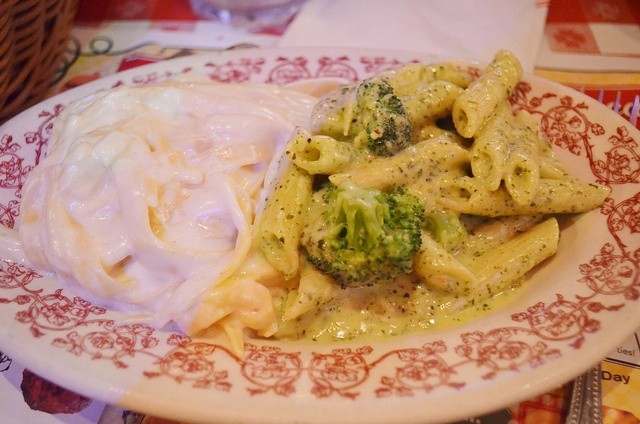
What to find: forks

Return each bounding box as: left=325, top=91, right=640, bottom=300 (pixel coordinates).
left=563, top=84, right=640, bottom=422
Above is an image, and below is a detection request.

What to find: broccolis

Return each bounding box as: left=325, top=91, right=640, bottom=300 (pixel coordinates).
left=311, top=180, right=426, bottom=289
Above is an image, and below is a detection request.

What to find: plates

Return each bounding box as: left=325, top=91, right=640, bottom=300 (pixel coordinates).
left=0, top=46, right=640, bottom=423
